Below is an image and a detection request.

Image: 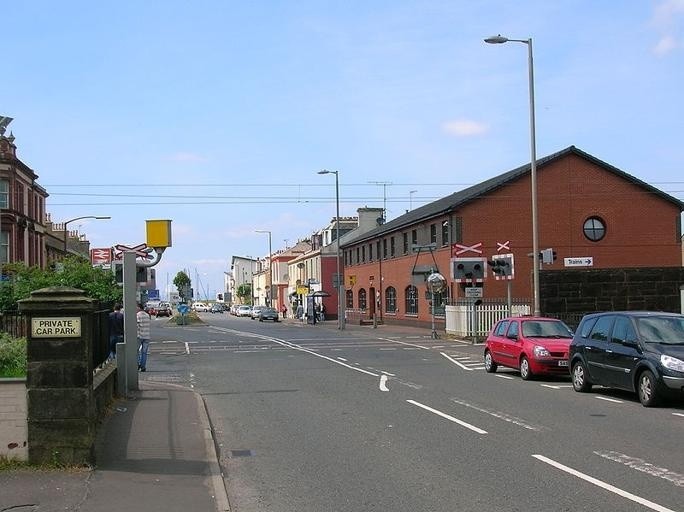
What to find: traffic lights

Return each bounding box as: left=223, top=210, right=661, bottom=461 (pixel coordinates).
left=549, top=248, right=557, bottom=264
left=452, top=259, right=487, bottom=280
left=485, top=256, right=513, bottom=276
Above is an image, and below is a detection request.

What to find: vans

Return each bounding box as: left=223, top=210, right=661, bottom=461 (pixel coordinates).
left=193, top=302, right=212, bottom=313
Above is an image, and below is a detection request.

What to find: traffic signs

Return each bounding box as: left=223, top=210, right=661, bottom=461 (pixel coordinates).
left=562, top=256, right=597, bottom=266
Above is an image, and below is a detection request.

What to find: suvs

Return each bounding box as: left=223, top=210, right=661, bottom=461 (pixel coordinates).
left=566, top=310, right=684, bottom=407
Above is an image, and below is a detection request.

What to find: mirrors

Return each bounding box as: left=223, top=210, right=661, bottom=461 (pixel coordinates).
left=425, top=272, right=448, bottom=296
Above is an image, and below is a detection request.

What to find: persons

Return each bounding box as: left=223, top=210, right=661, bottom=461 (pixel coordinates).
left=136, top=304, right=151, bottom=371
left=108, top=303, right=124, bottom=361
left=281, top=302, right=326, bottom=321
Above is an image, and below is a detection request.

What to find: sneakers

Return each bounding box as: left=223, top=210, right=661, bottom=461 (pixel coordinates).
left=138, top=362, right=141, bottom=370
left=141, top=365, right=146, bottom=372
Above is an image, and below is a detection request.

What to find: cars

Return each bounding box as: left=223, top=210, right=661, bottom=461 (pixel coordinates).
left=212, top=303, right=280, bottom=323
left=482, top=316, right=574, bottom=381
left=144, top=298, right=174, bottom=318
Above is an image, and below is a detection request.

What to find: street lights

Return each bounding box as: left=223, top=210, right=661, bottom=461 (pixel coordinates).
left=318, top=169, right=345, bottom=330
left=408, top=189, right=419, bottom=212
left=63, top=215, right=111, bottom=259
left=255, top=230, right=273, bottom=308
left=484, top=35, right=544, bottom=318
